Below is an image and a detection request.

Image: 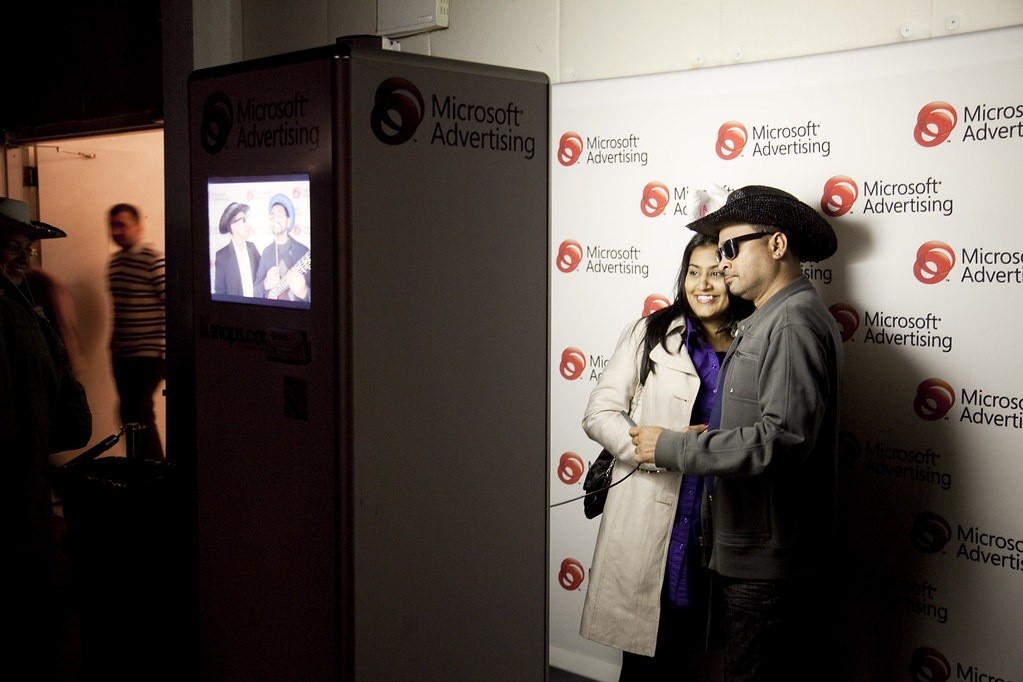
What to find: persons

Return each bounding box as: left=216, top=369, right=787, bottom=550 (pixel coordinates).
left=106, top=204, right=165, bottom=462
left=629, top=187, right=845, bottom=682
left=26, top=268, right=72, bottom=374
left=214, top=202, right=312, bottom=303
left=578, top=235, right=755, bottom=682
left=1, top=197, right=64, bottom=583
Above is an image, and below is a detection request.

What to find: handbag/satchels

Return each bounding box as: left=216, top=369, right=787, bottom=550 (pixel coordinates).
left=583, top=449, right=615, bottom=519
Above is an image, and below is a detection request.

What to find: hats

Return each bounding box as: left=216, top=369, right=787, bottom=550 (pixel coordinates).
left=219, top=202, right=249, bottom=234
left=685, top=185, right=837, bottom=262
left=1, top=195, right=68, bottom=240
left=267, top=194, right=295, bottom=232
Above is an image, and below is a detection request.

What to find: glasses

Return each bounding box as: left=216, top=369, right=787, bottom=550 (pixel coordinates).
left=231, top=217, right=246, bottom=223
left=1, top=242, right=38, bottom=256
left=715, top=231, right=775, bottom=263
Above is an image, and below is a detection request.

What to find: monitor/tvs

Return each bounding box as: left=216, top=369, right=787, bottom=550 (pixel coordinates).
left=206, top=172, right=312, bottom=308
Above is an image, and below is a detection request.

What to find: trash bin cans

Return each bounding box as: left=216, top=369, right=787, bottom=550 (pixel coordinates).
left=64, top=453, right=195, bottom=682
